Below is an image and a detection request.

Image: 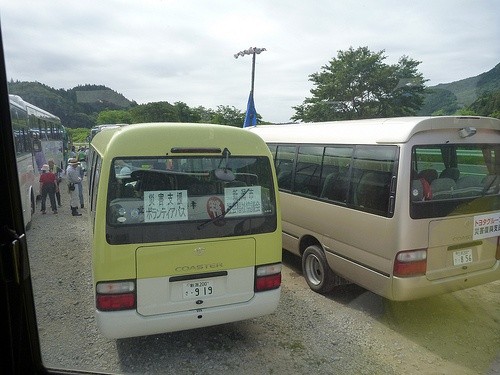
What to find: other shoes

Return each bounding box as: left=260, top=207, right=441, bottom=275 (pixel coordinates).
left=54, top=211, right=58, bottom=214
left=42, top=211, right=47, bottom=214
left=81, top=204, right=84, bottom=208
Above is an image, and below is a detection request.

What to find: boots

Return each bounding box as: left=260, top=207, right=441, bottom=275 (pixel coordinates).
left=72, top=208, right=82, bottom=216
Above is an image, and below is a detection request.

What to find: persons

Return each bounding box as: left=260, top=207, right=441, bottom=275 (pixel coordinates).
left=38, top=165, right=59, bottom=214
left=67, top=158, right=85, bottom=216
left=71, top=148, right=89, bottom=159
left=48, top=160, right=65, bottom=205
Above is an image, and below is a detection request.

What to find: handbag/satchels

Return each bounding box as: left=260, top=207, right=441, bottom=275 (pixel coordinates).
left=70, top=185, right=75, bottom=191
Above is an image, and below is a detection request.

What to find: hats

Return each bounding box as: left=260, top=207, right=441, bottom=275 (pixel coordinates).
left=68, top=158, right=78, bottom=164
left=41, top=165, right=50, bottom=171
left=75, top=158, right=80, bottom=163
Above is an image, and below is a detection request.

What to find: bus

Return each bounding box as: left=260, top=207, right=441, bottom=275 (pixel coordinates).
left=8, top=93, right=70, bottom=232
left=242, top=115, right=500, bottom=303
left=80, top=122, right=283, bottom=340
